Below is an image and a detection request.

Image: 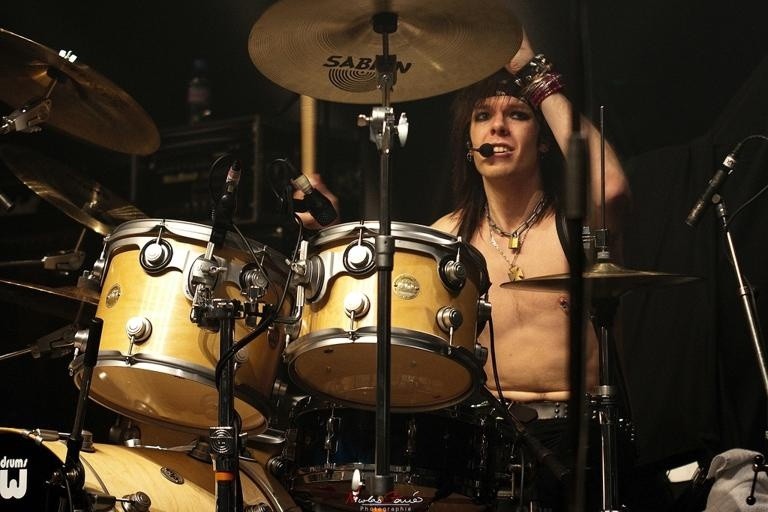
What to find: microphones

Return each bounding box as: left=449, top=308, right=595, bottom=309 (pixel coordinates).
left=212, top=159, right=242, bottom=223
left=291, top=169, right=337, bottom=228
left=462, top=142, right=495, bottom=158
left=684, top=144, right=737, bottom=228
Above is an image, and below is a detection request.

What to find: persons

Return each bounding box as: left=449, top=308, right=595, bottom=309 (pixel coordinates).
left=293, top=12, right=635, bottom=510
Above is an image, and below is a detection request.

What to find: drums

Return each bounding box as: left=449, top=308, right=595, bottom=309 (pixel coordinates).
left=282, top=221, right=492, bottom=413
left=281, top=395, right=496, bottom=502
left=67, top=219, right=294, bottom=438
left=1, top=425, right=299, bottom=511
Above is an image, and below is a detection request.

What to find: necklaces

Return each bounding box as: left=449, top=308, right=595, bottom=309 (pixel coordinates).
left=477, top=191, right=554, bottom=283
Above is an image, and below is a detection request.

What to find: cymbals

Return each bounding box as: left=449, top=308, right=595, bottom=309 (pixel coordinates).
left=1, top=280, right=100, bottom=306
left=248, top=1, right=523, bottom=104
left=0, top=29, right=160, bottom=157
left=500, top=264, right=698, bottom=292
left=4, top=157, right=152, bottom=239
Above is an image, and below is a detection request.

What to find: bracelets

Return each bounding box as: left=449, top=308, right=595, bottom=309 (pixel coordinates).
left=527, top=71, right=566, bottom=110
left=513, top=53, right=547, bottom=87
